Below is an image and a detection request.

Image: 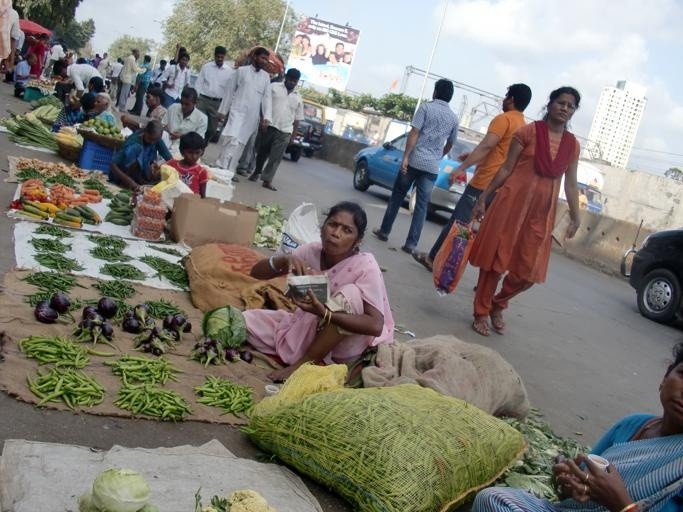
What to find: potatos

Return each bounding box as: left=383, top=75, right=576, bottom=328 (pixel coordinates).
left=15, top=200, right=102, bottom=228
left=104, top=197, right=133, bottom=226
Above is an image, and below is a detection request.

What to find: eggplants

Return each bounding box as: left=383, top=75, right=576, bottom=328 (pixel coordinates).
left=35, top=293, right=254, bottom=369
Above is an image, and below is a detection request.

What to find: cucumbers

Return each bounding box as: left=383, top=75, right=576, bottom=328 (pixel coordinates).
left=104, top=197, right=133, bottom=226
left=15, top=200, right=102, bottom=228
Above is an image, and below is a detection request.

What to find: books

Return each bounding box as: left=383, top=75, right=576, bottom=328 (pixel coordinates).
left=167, top=191, right=260, bottom=248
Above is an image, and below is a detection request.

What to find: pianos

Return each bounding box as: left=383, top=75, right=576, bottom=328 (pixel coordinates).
left=70, top=190, right=102, bottom=205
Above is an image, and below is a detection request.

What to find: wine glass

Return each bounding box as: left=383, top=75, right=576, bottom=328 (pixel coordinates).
left=582, top=472, right=589, bottom=482
left=583, top=484, right=587, bottom=494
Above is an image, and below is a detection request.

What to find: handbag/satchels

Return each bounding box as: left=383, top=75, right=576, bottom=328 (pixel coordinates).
left=327, top=312, right=331, bottom=327
left=268, top=256, right=278, bottom=276
left=320, top=308, right=328, bottom=320
left=619, top=503, right=637, bottom=511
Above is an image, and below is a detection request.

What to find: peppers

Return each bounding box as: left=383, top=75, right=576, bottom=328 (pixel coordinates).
left=194, top=374, right=256, bottom=417
left=20, top=335, right=91, bottom=368
left=21, top=227, right=189, bottom=319
left=103, top=353, right=194, bottom=423
left=238, top=385, right=526, bottom=512
left=26, top=367, right=106, bottom=409
left=87, top=347, right=117, bottom=357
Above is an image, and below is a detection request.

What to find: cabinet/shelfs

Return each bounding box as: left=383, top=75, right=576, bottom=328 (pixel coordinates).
left=215, top=119, right=223, bottom=131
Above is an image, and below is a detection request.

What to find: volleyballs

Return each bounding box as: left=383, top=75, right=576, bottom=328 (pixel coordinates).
left=35, top=293, right=254, bottom=369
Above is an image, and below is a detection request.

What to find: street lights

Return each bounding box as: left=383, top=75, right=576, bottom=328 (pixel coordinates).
left=431, top=219, right=477, bottom=299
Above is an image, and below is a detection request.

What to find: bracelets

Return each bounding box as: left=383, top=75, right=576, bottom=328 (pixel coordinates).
left=327, top=312, right=331, bottom=327
left=268, top=256, right=278, bottom=276
left=320, top=308, right=328, bottom=320
left=619, top=503, right=637, bottom=511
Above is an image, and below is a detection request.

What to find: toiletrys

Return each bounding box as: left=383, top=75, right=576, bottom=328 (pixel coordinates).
left=21, top=227, right=189, bottom=319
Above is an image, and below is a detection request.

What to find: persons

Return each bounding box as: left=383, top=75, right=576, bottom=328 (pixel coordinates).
left=409, top=82, right=532, bottom=271
left=465, top=338, right=682, bottom=511
left=108, top=120, right=174, bottom=192
left=1, top=2, right=20, bottom=70
left=238, top=200, right=396, bottom=384
left=371, top=78, right=460, bottom=255
left=161, top=89, right=208, bottom=160
left=158, top=44, right=304, bottom=191
left=469, top=86, right=583, bottom=337
left=290, top=34, right=353, bottom=83
left=90, top=53, right=125, bottom=87
left=3, top=29, right=74, bottom=99
left=166, top=132, right=208, bottom=198
left=118, top=49, right=167, bottom=117
left=120, top=86, right=168, bottom=131
left=51, top=59, right=116, bottom=130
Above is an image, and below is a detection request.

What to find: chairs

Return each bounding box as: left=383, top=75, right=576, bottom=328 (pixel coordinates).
left=372, top=226, right=418, bottom=254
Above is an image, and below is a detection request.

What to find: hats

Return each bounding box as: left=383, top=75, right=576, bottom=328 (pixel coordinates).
left=353, top=128, right=480, bottom=217
left=186, top=71, right=329, bottom=161
left=620, top=228, right=681, bottom=323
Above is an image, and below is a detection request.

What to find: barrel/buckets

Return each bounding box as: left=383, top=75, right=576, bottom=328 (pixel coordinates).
left=472, top=314, right=505, bottom=337
left=410, top=249, right=433, bottom=271
left=248, top=173, right=278, bottom=191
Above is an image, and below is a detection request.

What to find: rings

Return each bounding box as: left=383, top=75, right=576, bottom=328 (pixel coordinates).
left=583, top=484, right=587, bottom=494
left=582, top=472, right=589, bottom=482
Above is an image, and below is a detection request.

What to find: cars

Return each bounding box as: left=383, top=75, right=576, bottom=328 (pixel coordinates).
left=583, top=454, right=608, bottom=475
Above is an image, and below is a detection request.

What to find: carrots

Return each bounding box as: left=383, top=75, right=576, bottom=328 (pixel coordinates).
left=70, top=190, right=102, bottom=205
